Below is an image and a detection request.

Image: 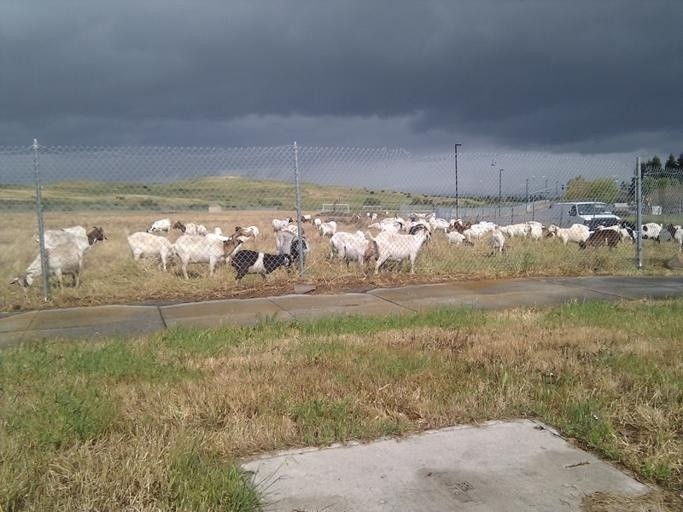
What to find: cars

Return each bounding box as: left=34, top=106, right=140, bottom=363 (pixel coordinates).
left=610, top=202, right=650, bottom=218
left=565, top=201, right=624, bottom=235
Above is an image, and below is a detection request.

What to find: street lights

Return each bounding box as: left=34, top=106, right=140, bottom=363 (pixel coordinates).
left=491, top=162, right=507, bottom=223
left=452, top=141, right=470, bottom=221
left=522, top=174, right=562, bottom=217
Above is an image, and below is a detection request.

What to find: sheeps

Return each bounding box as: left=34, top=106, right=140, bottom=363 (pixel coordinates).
left=9, top=240, right=83, bottom=296
left=125, top=210, right=683, bottom=288
left=33, top=226, right=109, bottom=253
